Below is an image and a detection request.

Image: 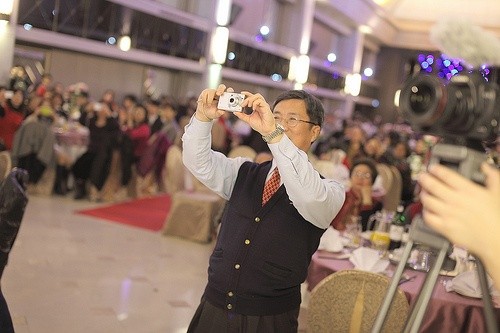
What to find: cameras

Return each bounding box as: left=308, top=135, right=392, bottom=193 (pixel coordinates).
left=217, top=91, right=246, bottom=113
left=397, top=68, right=500, bottom=149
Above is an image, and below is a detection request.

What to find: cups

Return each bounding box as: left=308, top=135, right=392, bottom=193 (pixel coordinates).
left=341, top=214, right=362, bottom=249
left=415, top=250, right=433, bottom=271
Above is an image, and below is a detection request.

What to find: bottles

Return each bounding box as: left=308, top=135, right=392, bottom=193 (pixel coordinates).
left=369, top=215, right=393, bottom=259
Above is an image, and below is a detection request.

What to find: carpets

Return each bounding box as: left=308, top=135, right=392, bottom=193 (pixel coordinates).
left=76, top=195, right=170, bottom=230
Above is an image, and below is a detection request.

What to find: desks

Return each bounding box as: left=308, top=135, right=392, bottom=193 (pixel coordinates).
left=305, top=239, right=500, bottom=333
left=49, top=118, right=90, bottom=185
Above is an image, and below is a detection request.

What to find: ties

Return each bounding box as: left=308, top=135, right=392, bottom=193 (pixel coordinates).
left=263, top=168, right=281, bottom=205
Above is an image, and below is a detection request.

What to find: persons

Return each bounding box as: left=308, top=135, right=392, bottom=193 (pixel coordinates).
left=419, top=160, right=500, bottom=294
left=136, top=93, right=198, bottom=197
left=182, top=84, right=346, bottom=333
left=313, top=117, right=426, bottom=229
left=0, top=74, right=151, bottom=201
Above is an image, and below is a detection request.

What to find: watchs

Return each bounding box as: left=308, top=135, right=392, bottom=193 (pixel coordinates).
left=262, top=123, right=284, bottom=142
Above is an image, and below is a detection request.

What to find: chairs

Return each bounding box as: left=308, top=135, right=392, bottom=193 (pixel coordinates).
left=304, top=270, right=409, bottom=333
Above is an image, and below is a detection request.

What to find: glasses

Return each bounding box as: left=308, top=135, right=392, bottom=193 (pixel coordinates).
left=274, top=114, right=316, bottom=128
left=353, top=171, right=372, bottom=182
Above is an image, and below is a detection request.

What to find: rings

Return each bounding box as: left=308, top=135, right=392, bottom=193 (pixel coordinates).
left=214, top=98, right=219, bottom=102
